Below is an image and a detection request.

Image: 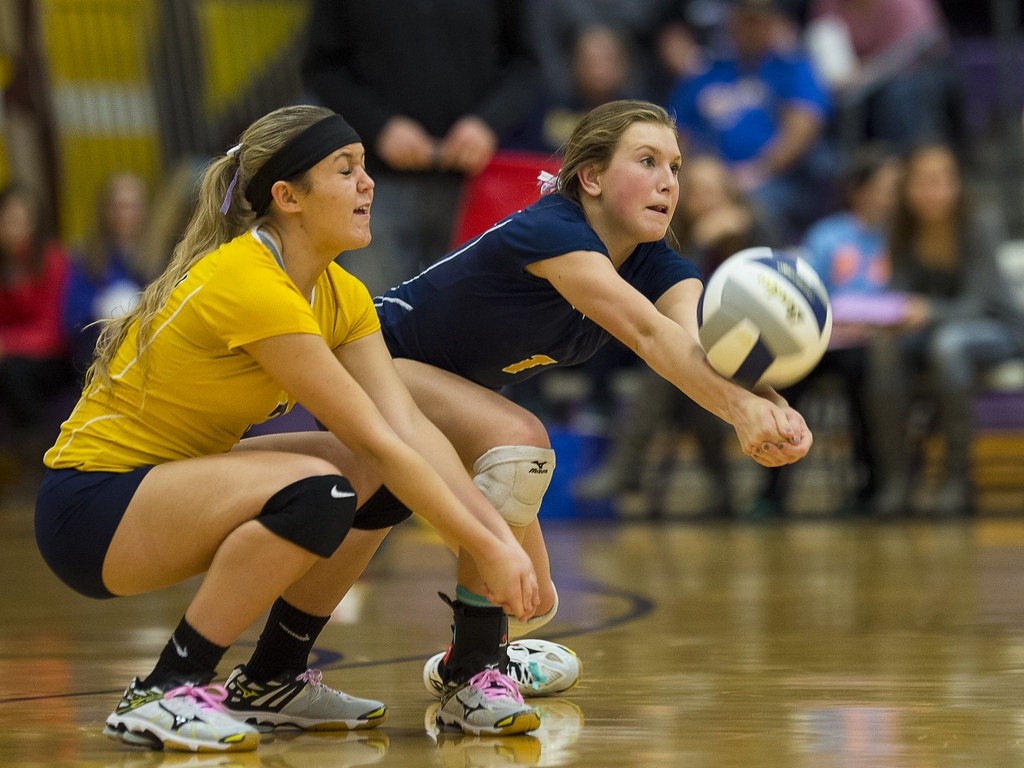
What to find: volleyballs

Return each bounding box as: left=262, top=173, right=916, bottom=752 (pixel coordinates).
left=696, top=245, right=832, bottom=388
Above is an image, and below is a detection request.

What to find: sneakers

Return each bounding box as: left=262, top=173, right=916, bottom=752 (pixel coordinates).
left=426, top=696, right=585, bottom=767
left=103, top=676, right=259, bottom=751
left=259, top=724, right=391, bottom=768
left=423, top=640, right=583, bottom=698
left=222, top=663, right=387, bottom=729
left=437, top=646, right=541, bottom=736
left=126, top=751, right=261, bottom=768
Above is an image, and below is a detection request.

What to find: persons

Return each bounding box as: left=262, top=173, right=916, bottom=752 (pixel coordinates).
left=31, top=102, right=542, bottom=752
left=312, top=100, right=816, bottom=698
left=0, top=1, right=1024, bottom=522
